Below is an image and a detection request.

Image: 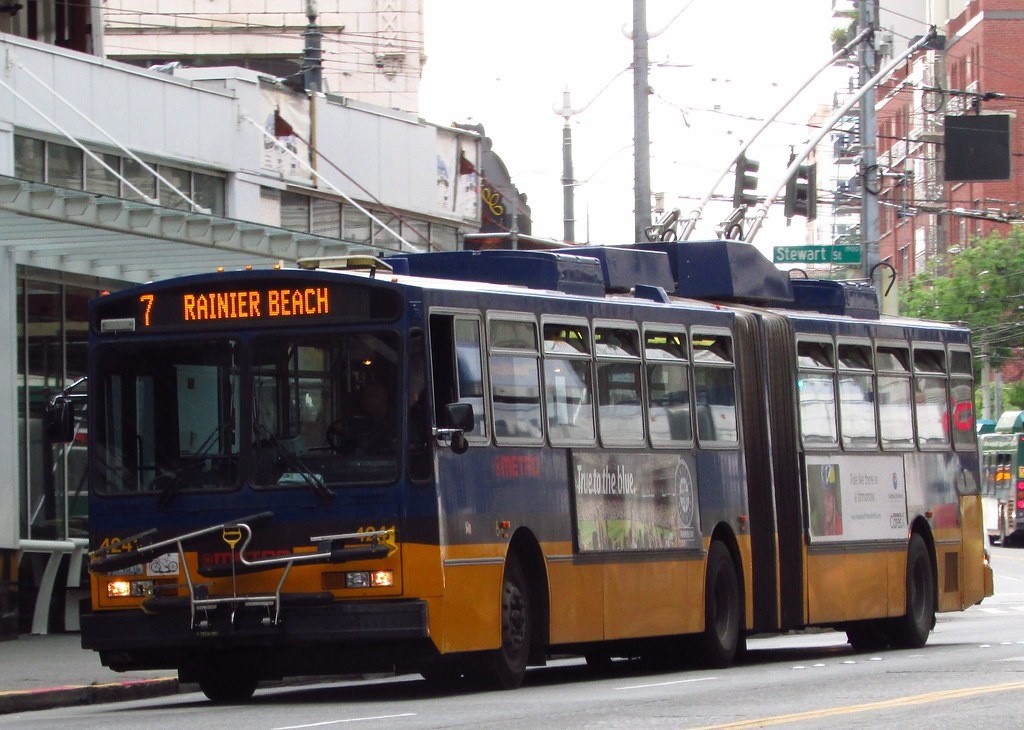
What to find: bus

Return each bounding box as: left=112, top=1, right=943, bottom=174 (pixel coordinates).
left=42, top=240, right=994, bottom=706
left=976, top=411, right=1024, bottom=546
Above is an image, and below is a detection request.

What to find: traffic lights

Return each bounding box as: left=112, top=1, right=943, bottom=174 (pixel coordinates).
left=784, top=161, right=810, bottom=217
left=733, top=154, right=758, bottom=207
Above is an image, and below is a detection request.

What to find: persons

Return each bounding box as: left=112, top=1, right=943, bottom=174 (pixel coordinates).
left=333, top=383, right=403, bottom=463
